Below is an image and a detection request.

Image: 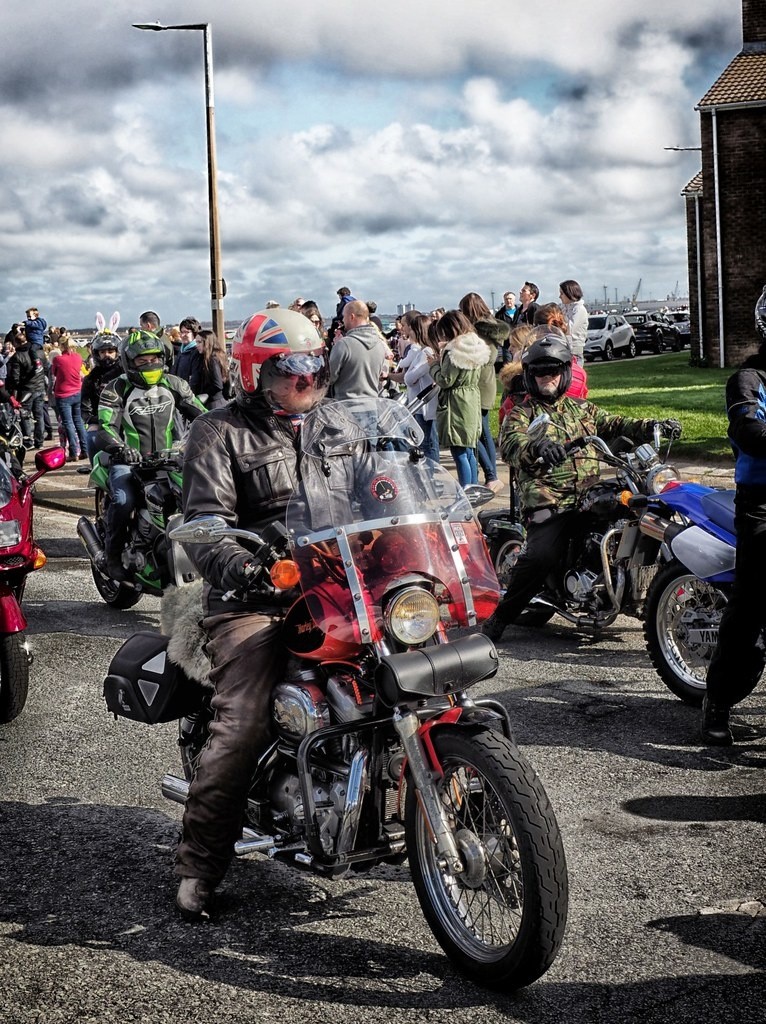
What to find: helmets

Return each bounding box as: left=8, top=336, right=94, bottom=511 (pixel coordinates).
left=83, top=327, right=124, bottom=374
left=517, top=334, right=576, bottom=402
left=126, top=331, right=171, bottom=388
left=229, top=307, right=327, bottom=392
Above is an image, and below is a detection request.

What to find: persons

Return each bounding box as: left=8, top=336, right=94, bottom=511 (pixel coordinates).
left=173, top=308, right=416, bottom=918
left=0, top=308, right=229, bottom=462
left=701, top=286, right=766, bottom=748
left=265, top=280, right=589, bottom=494
left=80, top=335, right=126, bottom=469
left=480, top=325, right=683, bottom=642
left=94, top=331, right=209, bottom=581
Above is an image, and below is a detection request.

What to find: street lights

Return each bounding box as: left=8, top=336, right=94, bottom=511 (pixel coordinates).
left=132, top=21, right=226, bottom=354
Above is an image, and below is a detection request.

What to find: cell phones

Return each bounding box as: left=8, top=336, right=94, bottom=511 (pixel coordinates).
left=423, top=349, right=434, bottom=358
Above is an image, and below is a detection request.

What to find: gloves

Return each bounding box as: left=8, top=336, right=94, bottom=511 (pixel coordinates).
left=221, top=552, right=257, bottom=594
left=660, top=418, right=683, bottom=438
left=536, top=440, right=569, bottom=468
left=121, top=446, right=143, bottom=464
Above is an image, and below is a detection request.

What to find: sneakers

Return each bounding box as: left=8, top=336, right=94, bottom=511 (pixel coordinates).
left=478, top=592, right=524, bottom=644
left=689, top=686, right=744, bottom=755
left=96, top=551, right=127, bottom=586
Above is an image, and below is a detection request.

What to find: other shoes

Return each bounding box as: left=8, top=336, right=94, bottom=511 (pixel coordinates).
left=176, top=872, right=216, bottom=916
left=35, top=365, right=44, bottom=374
left=66, top=453, right=89, bottom=462
left=44, top=432, right=52, bottom=440
left=487, top=480, right=505, bottom=494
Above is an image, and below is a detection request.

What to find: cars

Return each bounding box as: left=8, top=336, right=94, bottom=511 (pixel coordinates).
left=665, top=311, right=692, bottom=345
left=583, top=314, right=638, bottom=362
left=623, top=311, right=684, bottom=355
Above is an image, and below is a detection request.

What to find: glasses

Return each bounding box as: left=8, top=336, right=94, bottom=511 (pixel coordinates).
left=528, top=364, right=561, bottom=378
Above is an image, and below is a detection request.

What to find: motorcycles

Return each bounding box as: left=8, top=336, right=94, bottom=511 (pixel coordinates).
left=75, top=444, right=185, bottom=610
left=103, top=397, right=570, bottom=996
left=640, top=482, right=766, bottom=711
left=0, top=447, right=66, bottom=725
left=0, top=392, right=33, bottom=469
left=476, top=413, right=727, bottom=659
